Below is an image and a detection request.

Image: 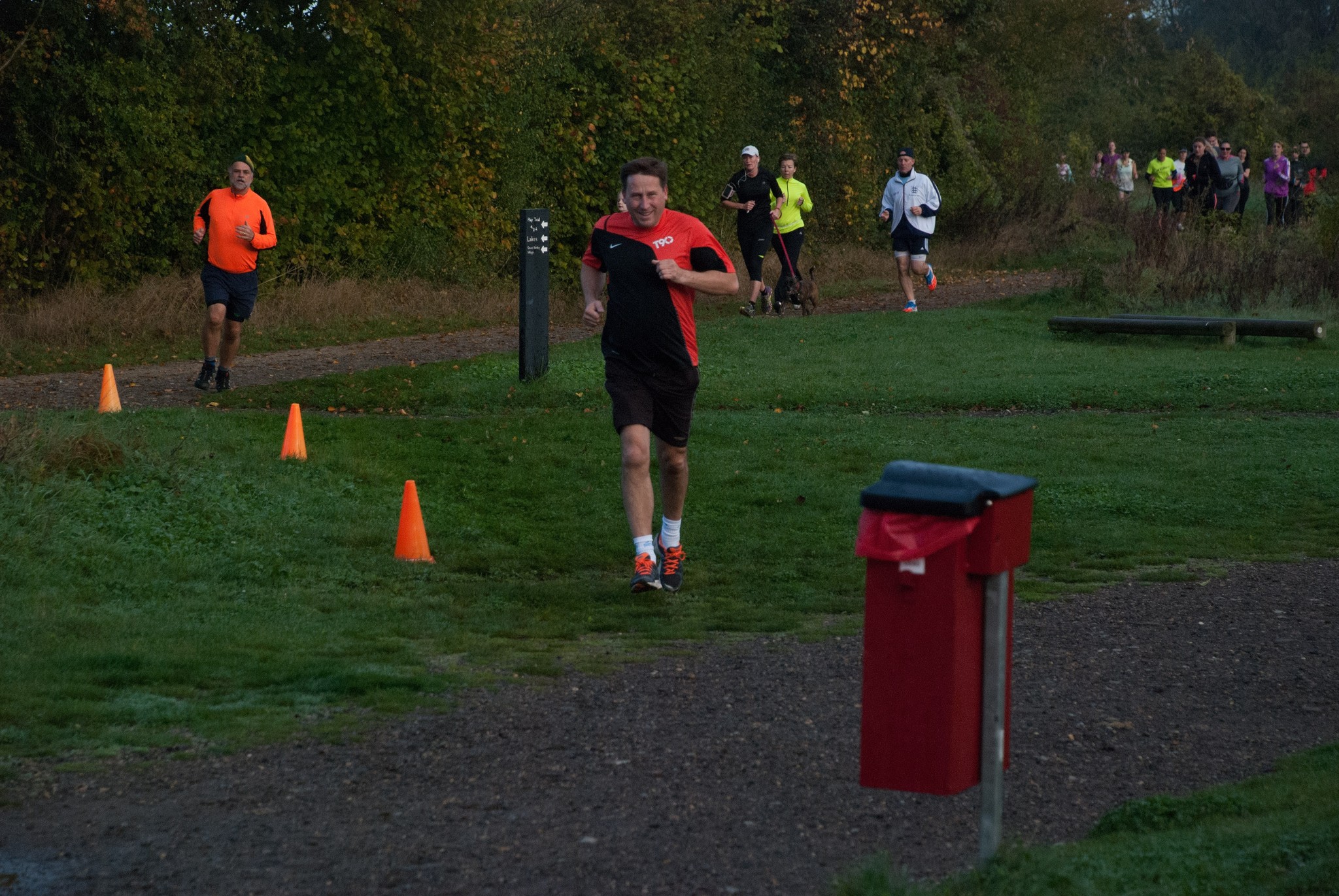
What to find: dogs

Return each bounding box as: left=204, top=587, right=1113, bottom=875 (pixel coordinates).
left=787, top=265, right=818, bottom=318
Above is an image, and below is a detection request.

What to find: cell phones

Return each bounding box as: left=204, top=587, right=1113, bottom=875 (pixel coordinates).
left=722, top=184, right=733, bottom=199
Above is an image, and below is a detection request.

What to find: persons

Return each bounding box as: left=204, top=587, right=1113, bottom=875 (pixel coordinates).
left=1284, top=146, right=1310, bottom=226
left=1145, top=146, right=1176, bottom=228
left=1233, top=147, right=1251, bottom=227
left=1204, top=134, right=1219, bottom=155
left=1174, top=147, right=1188, bottom=231
left=1298, top=142, right=1327, bottom=218
left=1055, top=152, right=1074, bottom=183
left=1099, top=141, right=1123, bottom=181
left=1262, top=141, right=1290, bottom=233
left=580, top=157, right=739, bottom=594
left=1089, top=151, right=1104, bottom=182
left=1111, top=147, right=1138, bottom=203
left=768, top=153, right=813, bottom=315
left=1185, top=137, right=1221, bottom=218
left=721, top=145, right=784, bottom=317
left=617, top=190, right=628, bottom=212
left=879, top=149, right=942, bottom=311
left=193, top=156, right=278, bottom=392
left=1215, top=141, right=1245, bottom=216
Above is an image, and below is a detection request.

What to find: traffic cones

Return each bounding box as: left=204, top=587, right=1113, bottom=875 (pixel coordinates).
left=98, top=363, right=122, bottom=415
left=397, top=479, right=438, bottom=566
left=280, top=402, right=308, bottom=461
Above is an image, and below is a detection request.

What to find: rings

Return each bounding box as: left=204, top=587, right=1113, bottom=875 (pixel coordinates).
left=243, top=233, right=245, bottom=236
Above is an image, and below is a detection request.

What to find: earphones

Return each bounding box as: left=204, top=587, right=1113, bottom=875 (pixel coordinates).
left=757, top=159, right=759, bottom=163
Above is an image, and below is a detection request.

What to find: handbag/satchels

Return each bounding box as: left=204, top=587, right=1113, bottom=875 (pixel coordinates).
left=1215, top=178, right=1234, bottom=190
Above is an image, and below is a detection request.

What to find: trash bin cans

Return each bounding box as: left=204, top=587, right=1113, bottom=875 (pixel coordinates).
left=860, top=459, right=1038, bottom=801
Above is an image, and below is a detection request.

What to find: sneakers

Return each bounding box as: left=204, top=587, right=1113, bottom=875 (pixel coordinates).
left=214, top=368, right=235, bottom=393
left=902, top=301, right=918, bottom=313
left=653, top=532, right=687, bottom=592
left=790, top=292, right=802, bottom=310
left=773, top=301, right=786, bottom=318
left=739, top=303, right=755, bottom=319
left=924, top=262, right=937, bottom=291
left=193, top=360, right=217, bottom=391
left=760, top=285, right=773, bottom=315
left=629, top=552, right=662, bottom=593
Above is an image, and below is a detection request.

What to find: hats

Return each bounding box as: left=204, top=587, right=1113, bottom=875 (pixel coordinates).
left=1178, top=145, right=1187, bottom=153
left=740, top=145, right=760, bottom=157
left=897, top=147, right=914, bottom=158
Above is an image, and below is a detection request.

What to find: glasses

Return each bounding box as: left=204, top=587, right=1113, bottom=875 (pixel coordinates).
left=1220, top=147, right=1231, bottom=151
